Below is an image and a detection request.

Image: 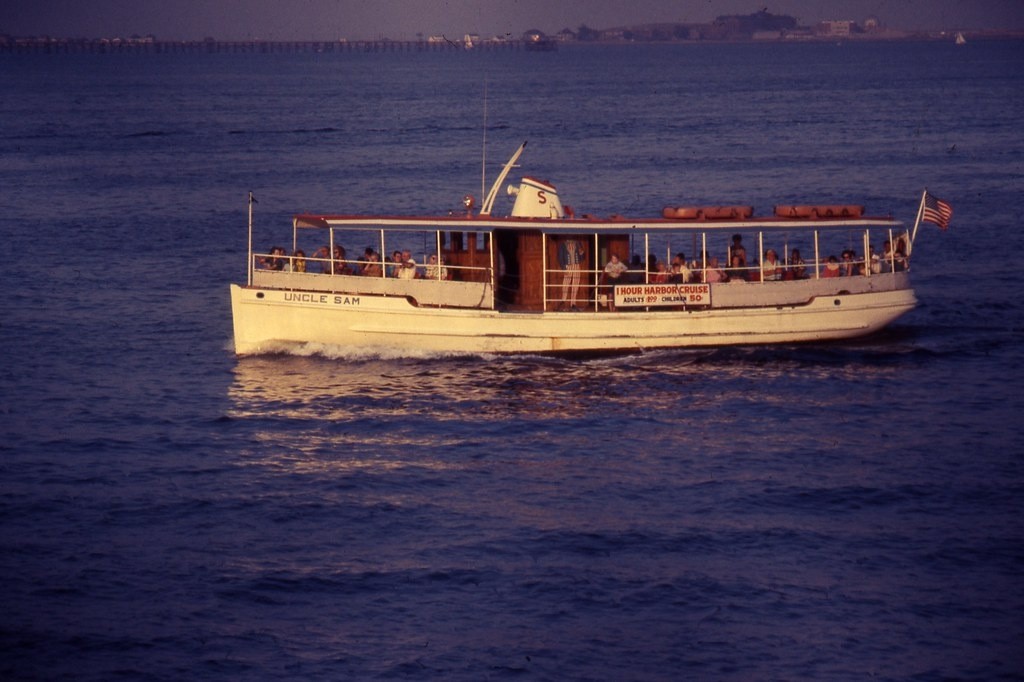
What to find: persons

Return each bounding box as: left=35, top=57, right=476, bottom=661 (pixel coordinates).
left=671, top=253, right=702, bottom=284
left=334, top=245, right=352, bottom=275
left=883, top=238, right=909, bottom=271
left=700, top=251, right=728, bottom=282
left=605, top=254, right=627, bottom=312
left=842, top=246, right=881, bottom=276
left=762, top=250, right=783, bottom=279
left=296, top=250, right=306, bottom=272
left=824, top=256, right=841, bottom=278
left=424, top=253, right=448, bottom=280
left=648, top=253, right=668, bottom=283
left=358, top=248, right=382, bottom=277
left=624, top=256, right=643, bottom=283
left=782, top=250, right=807, bottom=279
left=726, top=234, right=748, bottom=279
left=260, top=247, right=289, bottom=271
left=385, top=250, right=415, bottom=278
left=311, top=246, right=331, bottom=273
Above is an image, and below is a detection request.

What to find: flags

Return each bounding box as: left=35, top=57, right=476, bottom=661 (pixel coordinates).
left=922, top=193, right=952, bottom=231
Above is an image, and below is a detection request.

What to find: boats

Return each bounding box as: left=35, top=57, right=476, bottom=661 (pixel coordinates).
left=775, top=204, right=866, bottom=219
left=230, top=71, right=924, bottom=362
left=662, top=204, right=754, bottom=220
